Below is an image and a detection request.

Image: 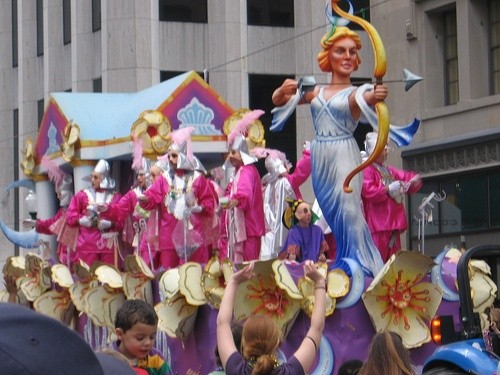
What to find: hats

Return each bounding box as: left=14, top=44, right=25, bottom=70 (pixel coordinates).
left=95, top=158, right=116, bottom=189
left=365, top=132, right=390, bottom=155
left=228, top=133, right=259, bottom=166
left=260, top=154, right=292, bottom=183
left=155, top=157, right=172, bottom=186
left=0, top=302, right=135, bottom=375
left=133, top=157, right=153, bottom=188
left=282, top=198, right=320, bottom=228
left=169, top=126, right=208, bottom=175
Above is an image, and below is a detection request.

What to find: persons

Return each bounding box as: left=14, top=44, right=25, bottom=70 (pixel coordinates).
left=0, top=301, right=137, bottom=375
left=361, top=132, right=422, bottom=263
left=272, top=25, right=388, bottom=278
left=110, top=299, right=173, bottom=375
left=278, top=198, right=330, bottom=261
left=216, top=259, right=326, bottom=374
left=22, top=158, right=78, bottom=274
left=65, top=159, right=121, bottom=270
left=95, top=109, right=311, bottom=268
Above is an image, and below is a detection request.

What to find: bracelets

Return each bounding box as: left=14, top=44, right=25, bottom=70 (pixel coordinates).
left=315, top=287, right=325, bottom=289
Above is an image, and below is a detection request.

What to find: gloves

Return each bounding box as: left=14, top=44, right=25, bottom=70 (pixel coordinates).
left=191, top=201, right=202, bottom=214
left=388, top=180, right=401, bottom=193
left=79, top=216, right=93, bottom=228
left=98, top=219, right=111, bottom=230
